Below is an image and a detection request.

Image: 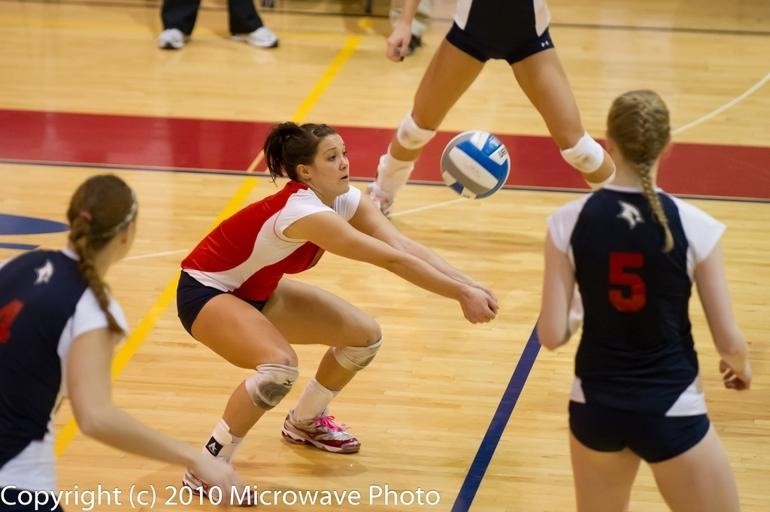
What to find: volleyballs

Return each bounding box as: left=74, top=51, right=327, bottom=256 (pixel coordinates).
left=441, top=130, right=510, bottom=201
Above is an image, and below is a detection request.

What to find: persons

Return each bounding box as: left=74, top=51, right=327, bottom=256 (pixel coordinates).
left=0, top=174, right=245, bottom=512
left=176, top=121, right=500, bottom=507
left=536, top=90, right=751, bottom=512
left=364, top=0, right=617, bottom=216
left=158, top=0, right=279, bottom=49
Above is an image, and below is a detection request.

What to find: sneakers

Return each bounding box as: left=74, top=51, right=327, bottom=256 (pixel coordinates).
left=282, top=411, right=362, bottom=454
left=363, top=184, right=391, bottom=217
left=397, top=33, right=423, bottom=56
left=157, top=26, right=192, bottom=50
left=181, top=465, right=258, bottom=507
left=230, top=27, right=278, bottom=48
